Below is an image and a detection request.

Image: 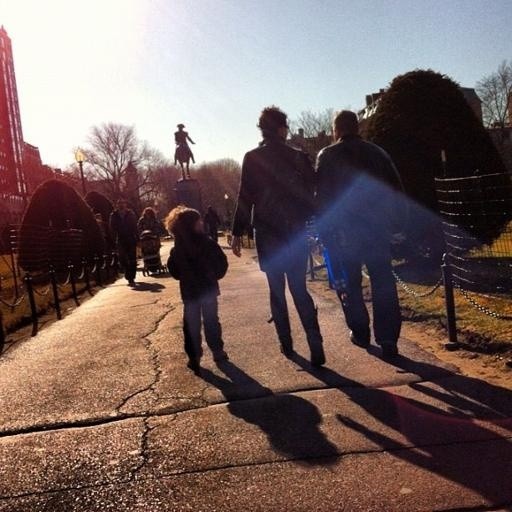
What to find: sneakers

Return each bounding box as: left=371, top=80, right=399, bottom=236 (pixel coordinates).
left=187, top=359, right=201, bottom=368
left=212, top=351, right=228, bottom=362
left=127, top=280, right=136, bottom=287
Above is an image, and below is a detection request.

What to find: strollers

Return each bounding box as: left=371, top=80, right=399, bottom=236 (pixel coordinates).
left=140, top=230, right=168, bottom=277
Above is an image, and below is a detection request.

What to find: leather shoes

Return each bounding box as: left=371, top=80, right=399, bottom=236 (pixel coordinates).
left=349, top=330, right=397, bottom=350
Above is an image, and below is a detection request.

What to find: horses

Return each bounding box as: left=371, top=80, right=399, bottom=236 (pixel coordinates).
left=176, top=134, right=191, bottom=180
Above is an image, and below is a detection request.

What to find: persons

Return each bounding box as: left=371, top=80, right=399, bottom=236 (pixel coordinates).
left=313, top=108, right=412, bottom=358
left=229, top=103, right=328, bottom=369
left=137, top=207, right=164, bottom=274
left=160, top=202, right=229, bottom=370
left=107, top=195, right=140, bottom=288
left=173, top=122, right=197, bottom=166
left=203, top=203, right=222, bottom=243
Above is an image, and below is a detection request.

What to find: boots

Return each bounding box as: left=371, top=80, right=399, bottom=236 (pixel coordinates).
left=268, top=312, right=292, bottom=356
left=300, top=310, right=326, bottom=366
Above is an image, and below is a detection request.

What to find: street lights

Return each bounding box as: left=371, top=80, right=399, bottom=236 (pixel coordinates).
left=76, top=151, right=87, bottom=196
left=225, top=193, right=228, bottom=232
